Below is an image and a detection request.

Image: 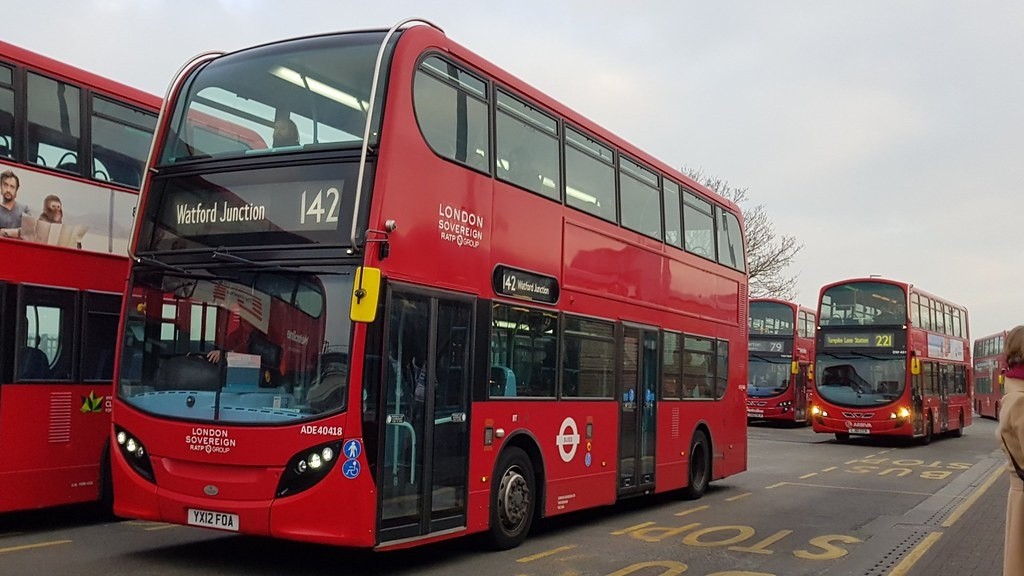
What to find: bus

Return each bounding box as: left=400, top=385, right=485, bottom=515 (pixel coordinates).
left=0, top=37, right=277, bottom=531
left=714, top=298, right=816, bottom=425
left=972, top=329, right=1012, bottom=420
left=107, top=19, right=748, bottom=552
left=809, top=273, right=973, bottom=445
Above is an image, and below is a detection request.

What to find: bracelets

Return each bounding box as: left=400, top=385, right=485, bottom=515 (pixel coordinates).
left=4, top=228, right=8, bottom=238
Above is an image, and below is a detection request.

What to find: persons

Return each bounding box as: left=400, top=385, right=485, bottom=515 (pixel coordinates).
left=661, top=347, right=727, bottom=399
left=273, top=119, right=301, bottom=147
left=206, top=299, right=271, bottom=364
left=0, top=170, right=34, bottom=239
left=996, top=325, right=1024, bottom=576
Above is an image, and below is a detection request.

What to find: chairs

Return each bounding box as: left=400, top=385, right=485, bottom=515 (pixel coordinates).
left=692, top=245, right=707, bottom=257
left=113, top=166, right=141, bottom=187
left=750, top=325, right=761, bottom=334
left=654, top=231, right=672, bottom=244
left=844, top=314, right=859, bottom=325
left=777, top=326, right=788, bottom=335
left=55, top=152, right=79, bottom=173
left=673, top=239, right=690, bottom=250
left=491, top=365, right=517, bottom=397
left=828, top=314, right=842, bottom=325
